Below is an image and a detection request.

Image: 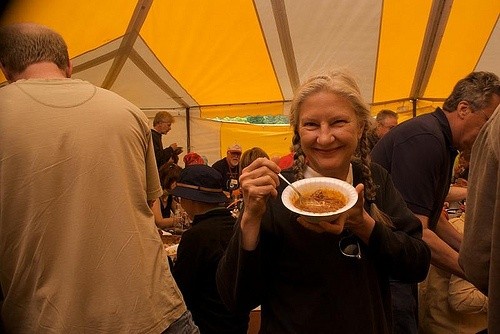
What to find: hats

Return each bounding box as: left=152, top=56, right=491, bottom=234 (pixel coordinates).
left=227, top=143, right=243, bottom=154
left=201, top=155, right=208, bottom=166
left=182, top=152, right=203, bottom=165
left=171, top=164, right=233, bottom=203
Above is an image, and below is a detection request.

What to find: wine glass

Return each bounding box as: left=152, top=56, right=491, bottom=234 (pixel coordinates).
left=232, top=191, right=240, bottom=214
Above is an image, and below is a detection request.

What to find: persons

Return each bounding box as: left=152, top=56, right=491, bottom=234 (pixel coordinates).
left=362, top=71, right=500, bottom=334
left=0, top=26, right=199, bottom=333
left=216, top=71, right=431, bottom=333
left=150, top=111, right=271, bottom=334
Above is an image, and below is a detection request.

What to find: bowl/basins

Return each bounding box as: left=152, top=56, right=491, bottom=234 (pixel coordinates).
left=281, top=176, right=358, bottom=224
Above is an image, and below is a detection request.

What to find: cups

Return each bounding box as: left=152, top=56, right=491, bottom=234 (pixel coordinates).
left=173, top=209, right=190, bottom=233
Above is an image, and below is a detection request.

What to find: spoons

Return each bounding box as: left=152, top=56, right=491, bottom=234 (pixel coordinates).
left=264, top=156, right=320, bottom=206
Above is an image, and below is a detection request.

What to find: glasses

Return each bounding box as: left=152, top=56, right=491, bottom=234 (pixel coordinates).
left=378, top=122, right=395, bottom=130
left=229, top=153, right=241, bottom=157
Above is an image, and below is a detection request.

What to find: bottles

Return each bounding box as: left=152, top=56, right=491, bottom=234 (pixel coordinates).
left=174, top=203, right=182, bottom=215
